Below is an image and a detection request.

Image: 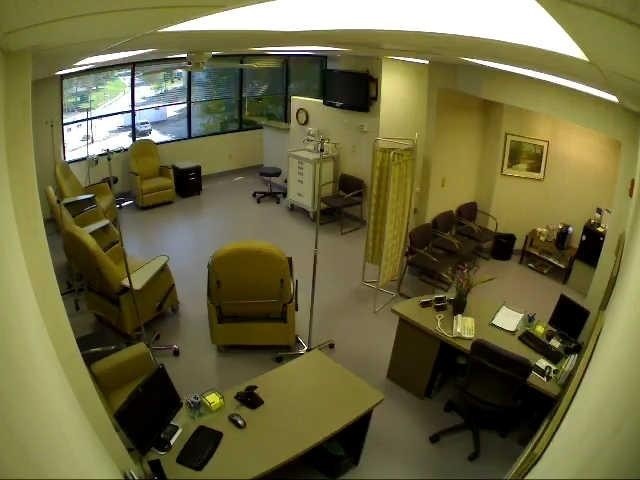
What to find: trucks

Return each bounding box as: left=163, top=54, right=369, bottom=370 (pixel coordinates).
left=123, top=103, right=167, bottom=127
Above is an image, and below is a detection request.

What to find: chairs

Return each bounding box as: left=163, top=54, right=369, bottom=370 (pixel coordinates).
left=205, top=239, right=300, bottom=353
left=395, top=199, right=499, bottom=297
left=45, top=159, right=181, bottom=346
left=128, top=138, right=176, bottom=209
left=318, top=172, right=368, bottom=235
left=429, top=339, right=533, bottom=461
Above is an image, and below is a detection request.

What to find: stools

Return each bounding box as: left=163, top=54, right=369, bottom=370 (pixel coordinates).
left=252, top=166, right=285, bottom=204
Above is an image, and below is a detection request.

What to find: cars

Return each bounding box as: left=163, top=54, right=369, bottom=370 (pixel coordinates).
left=128, top=77, right=144, bottom=86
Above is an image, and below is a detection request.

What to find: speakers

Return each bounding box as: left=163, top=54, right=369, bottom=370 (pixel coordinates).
left=576, top=218, right=607, bottom=267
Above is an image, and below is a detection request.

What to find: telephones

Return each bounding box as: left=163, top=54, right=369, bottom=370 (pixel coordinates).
left=452, top=313, right=475, bottom=339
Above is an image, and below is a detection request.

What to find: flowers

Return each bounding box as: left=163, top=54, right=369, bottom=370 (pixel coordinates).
left=440, top=262, right=496, bottom=296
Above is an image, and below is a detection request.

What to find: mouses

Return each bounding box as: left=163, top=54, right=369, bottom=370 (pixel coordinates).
left=228, top=413, right=247, bottom=428
left=544, top=366, right=551, bottom=376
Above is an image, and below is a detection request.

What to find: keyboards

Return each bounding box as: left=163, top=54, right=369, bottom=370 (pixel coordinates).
left=176, top=424, right=224, bottom=471
left=518, top=329, right=564, bottom=364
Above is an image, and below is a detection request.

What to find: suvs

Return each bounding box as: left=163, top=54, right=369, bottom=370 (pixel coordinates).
left=135, top=120, right=152, bottom=134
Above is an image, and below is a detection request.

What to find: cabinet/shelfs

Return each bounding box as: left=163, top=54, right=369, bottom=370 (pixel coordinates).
left=286, top=148, right=338, bottom=222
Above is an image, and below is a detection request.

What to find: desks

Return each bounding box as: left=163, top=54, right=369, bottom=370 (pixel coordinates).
left=385, top=290, right=567, bottom=448
left=140, top=348, right=384, bottom=479
left=518, top=228, right=578, bottom=285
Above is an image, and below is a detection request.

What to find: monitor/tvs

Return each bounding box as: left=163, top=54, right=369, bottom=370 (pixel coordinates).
left=113, top=363, right=184, bottom=455
left=548, top=293, right=591, bottom=340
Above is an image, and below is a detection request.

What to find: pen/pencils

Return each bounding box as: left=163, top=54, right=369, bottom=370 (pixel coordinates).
left=528, top=313, right=537, bottom=323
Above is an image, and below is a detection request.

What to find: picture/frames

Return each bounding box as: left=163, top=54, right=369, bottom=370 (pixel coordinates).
left=501, top=131, right=550, bottom=181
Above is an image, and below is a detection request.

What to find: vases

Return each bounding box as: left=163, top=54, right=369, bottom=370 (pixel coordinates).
left=452, top=294, right=467, bottom=316
